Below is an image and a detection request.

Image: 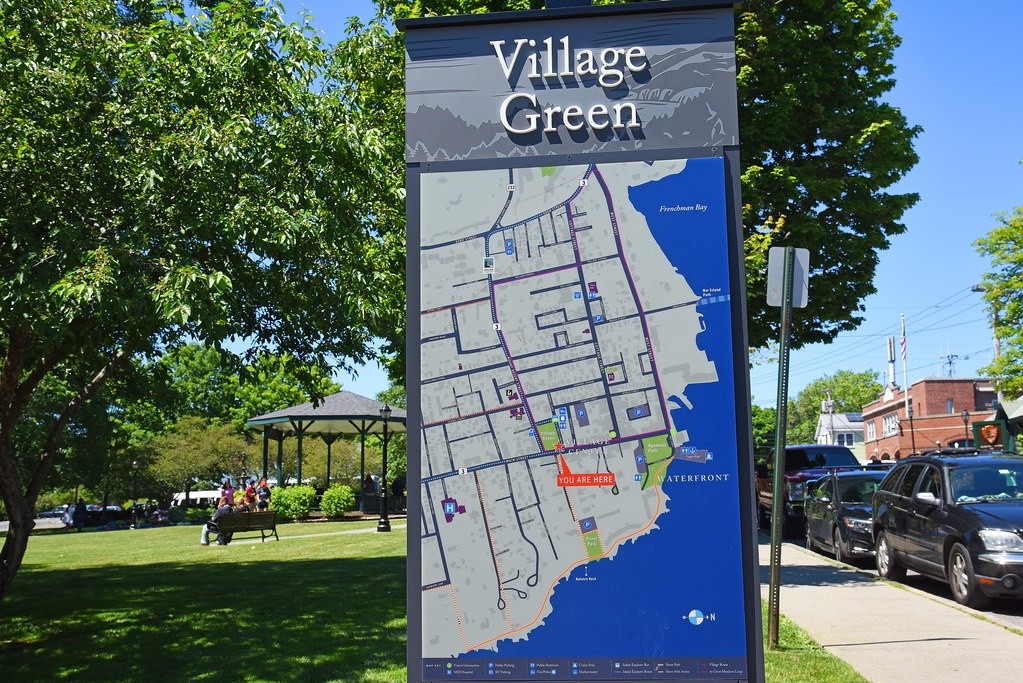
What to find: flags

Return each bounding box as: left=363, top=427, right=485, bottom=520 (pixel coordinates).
left=900, top=322, right=906, bottom=362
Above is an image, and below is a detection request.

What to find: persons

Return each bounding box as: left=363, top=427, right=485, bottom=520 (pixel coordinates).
left=64, top=498, right=86, bottom=532
left=200, top=477, right=271, bottom=546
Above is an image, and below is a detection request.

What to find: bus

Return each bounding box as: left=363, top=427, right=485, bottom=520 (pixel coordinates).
left=170, top=490, right=223, bottom=509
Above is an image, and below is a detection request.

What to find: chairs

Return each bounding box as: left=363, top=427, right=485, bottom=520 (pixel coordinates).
left=986, top=473, right=1006, bottom=492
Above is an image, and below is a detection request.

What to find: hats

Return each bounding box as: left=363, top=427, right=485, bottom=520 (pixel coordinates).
left=246, top=479, right=255, bottom=484
left=261, top=481, right=266, bottom=487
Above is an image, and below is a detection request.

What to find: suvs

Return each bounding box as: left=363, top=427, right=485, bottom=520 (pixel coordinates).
left=871, top=444, right=1023, bottom=608
left=754, top=444, right=886, bottom=538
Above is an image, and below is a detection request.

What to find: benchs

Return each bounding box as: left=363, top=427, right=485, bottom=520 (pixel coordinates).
left=205, top=508, right=280, bottom=546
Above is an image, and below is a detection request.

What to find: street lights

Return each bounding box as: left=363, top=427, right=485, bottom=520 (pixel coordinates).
left=959, top=409, right=970, bottom=450
left=907, top=406, right=917, bottom=456
left=377, top=403, right=392, bottom=533
left=130, top=461, right=140, bottom=530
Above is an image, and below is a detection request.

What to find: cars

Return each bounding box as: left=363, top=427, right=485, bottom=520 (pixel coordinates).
left=804, top=463, right=898, bottom=564
left=36, top=502, right=155, bottom=527
